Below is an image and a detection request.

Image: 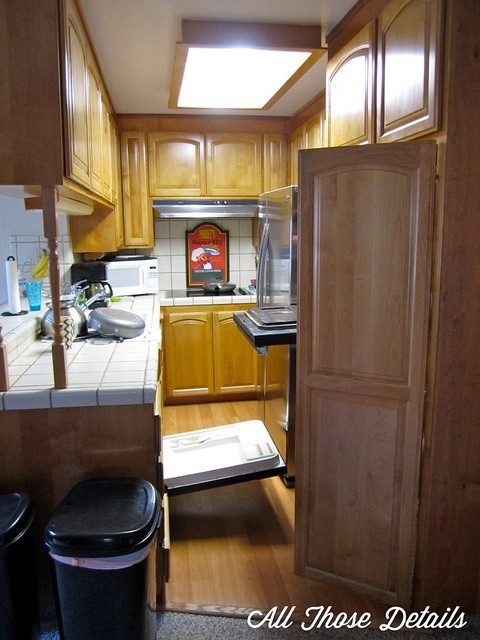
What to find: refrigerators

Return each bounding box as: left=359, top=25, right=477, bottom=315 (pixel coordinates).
left=255, top=186, right=296, bottom=488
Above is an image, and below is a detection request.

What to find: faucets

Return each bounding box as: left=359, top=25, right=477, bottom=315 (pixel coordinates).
left=71, top=279, right=106, bottom=312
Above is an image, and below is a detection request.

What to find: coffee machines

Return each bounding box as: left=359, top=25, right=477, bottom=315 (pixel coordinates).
left=70, top=262, right=113, bottom=308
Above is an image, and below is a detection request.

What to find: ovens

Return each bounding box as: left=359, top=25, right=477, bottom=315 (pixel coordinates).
left=233, top=303, right=298, bottom=354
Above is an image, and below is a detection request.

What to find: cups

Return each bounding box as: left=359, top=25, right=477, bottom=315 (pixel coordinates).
left=24, top=281, right=42, bottom=311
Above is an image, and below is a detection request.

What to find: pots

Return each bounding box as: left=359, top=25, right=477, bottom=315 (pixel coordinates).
left=202, top=277, right=236, bottom=295
left=74, top=307, right=146, bottom=341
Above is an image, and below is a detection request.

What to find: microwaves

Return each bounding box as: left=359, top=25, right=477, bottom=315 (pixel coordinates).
left=101, top=255, right=159, bottom=296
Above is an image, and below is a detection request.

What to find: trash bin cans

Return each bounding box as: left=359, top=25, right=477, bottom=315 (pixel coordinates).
left=44, top=477, right=163, bottom=639
left=0, top=491, right=38, bottom=640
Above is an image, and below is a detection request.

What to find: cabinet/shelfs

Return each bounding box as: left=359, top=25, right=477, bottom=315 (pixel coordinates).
left=145, top=114, right=288, bottom=202
left=0, top=3, right=118, bottom=214
left=323, top=0, right=479, bottom=152
left=67, top=114, right=152, bottom=251
left=164, top=304, right=261, bottom=401
left=284, top=93, right=329, bottom=211
left=0, top=308, right=171, bottom=616
left=292, top=138, right=478, bottom=618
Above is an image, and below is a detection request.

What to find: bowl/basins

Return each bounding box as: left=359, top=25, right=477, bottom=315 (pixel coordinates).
left=40, top=300, right=87, bottom=336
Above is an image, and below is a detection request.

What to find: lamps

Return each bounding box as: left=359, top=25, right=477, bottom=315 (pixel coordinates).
left=164, top=20, right=327, bottom=111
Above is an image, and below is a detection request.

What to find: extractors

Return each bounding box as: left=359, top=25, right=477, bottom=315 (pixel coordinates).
left=153, top=198, right=258, bottom=220
